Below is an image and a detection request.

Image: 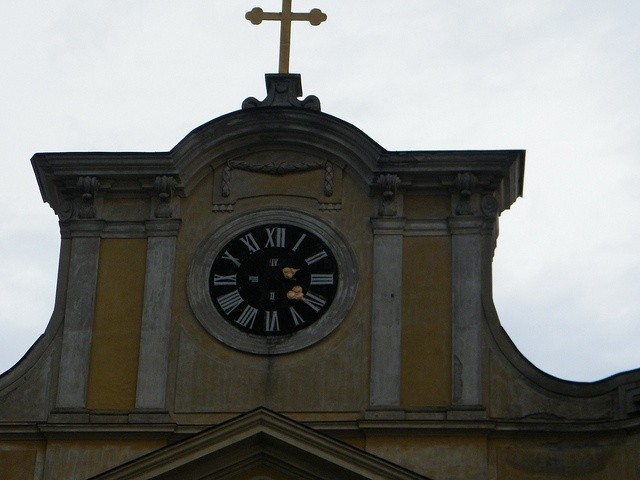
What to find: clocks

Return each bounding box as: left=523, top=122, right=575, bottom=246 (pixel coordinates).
left=185, top=207, right=358, bottom=354
